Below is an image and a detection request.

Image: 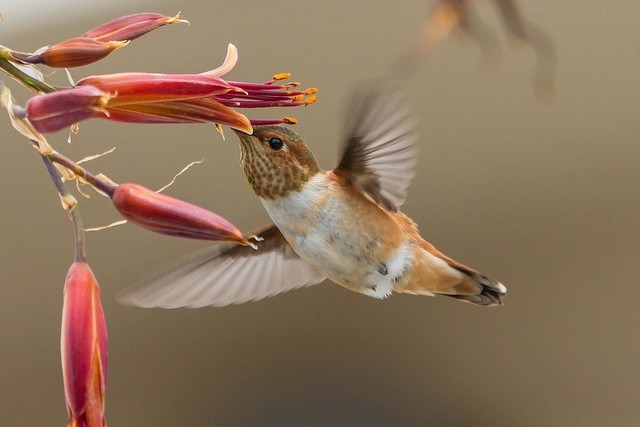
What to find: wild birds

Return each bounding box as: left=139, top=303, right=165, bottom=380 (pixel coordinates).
left=113, top=77, right=507, bottom=310
